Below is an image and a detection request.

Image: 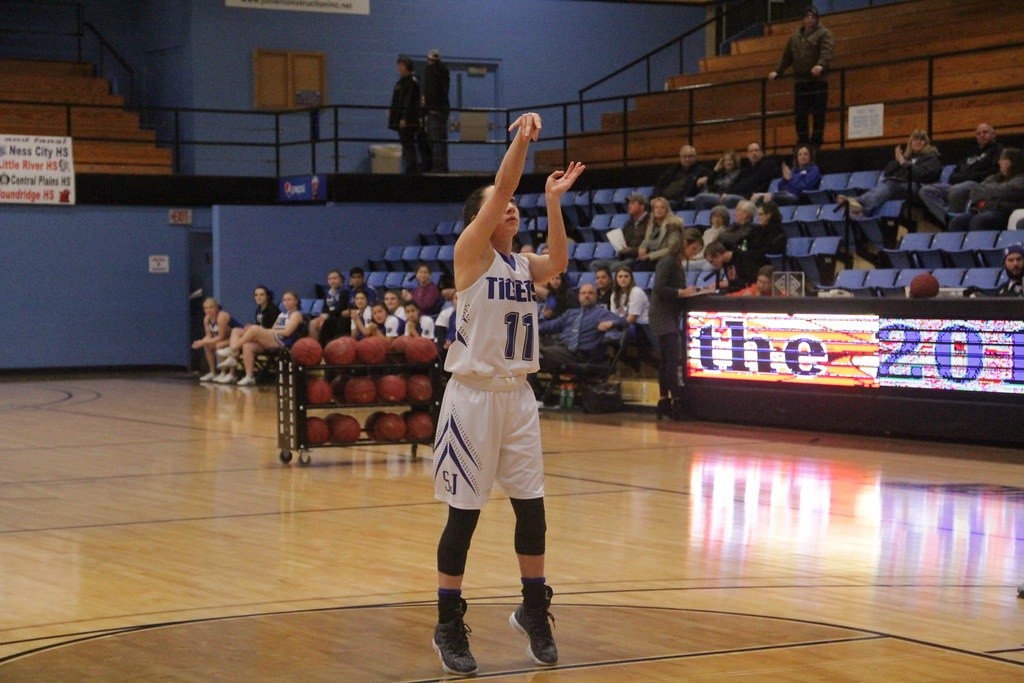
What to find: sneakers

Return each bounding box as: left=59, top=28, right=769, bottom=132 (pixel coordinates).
left=212, top=375, right=226, bottom=381
left=217, top=356, right=237, bottom=368
left=218, top=373, right=237, bottom=383
left=508, top=603, right=558, bottom=665
left=217, top=347, right=231, bottom=357
left=432, top=617, right=478, bottom=675
left=199, top=372, right=215, bottom=381
left=237, top=376, right=255, bottom=385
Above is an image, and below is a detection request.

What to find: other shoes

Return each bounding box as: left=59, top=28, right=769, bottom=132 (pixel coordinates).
left=837, top=194, right=862, bottom=211
left=533, top=388, right=545, bottom=400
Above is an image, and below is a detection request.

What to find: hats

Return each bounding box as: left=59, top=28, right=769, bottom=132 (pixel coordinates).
left=625, top=193, right=647, bottom=205
left=1001, top=244, right=1024, bottom=261
left=804, top=5, right=818, bottom=18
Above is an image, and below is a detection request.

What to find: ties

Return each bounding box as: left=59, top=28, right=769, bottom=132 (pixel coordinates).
left=568, top=312, right=584, bottom=351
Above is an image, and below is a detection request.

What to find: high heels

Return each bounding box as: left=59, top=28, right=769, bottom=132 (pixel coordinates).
left=655, top=398, right=675, bottom=419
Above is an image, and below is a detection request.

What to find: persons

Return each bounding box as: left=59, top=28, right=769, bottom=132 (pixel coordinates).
left=590, top=193, right=684, bottom=271
left=387, top=49, right=450, bottom=176
left=192, top=263, right=457, bottom=387
left=520, top=210, right=650, bottom=407
left=769, top=6, right=836, bottom=150
left=648, top=228, right=704, bottom=422
left=650, top=142, right=781, bottom=208
left=703, top=241, right=758, bottom=290
left=995, top=244, right=1024, bottom=297
left=432, top=113, right=586, bottom=674
left=726, top=264, right=798, bottom=296
left=837, top=130, right=942, bottom=218
left=749, top=144, right=822, bottom=208
left=918, top=123, right=1004, bottom=225
left=948, top=148, right=1024, bottom=230
left=696, top=200, right=788, bottom=265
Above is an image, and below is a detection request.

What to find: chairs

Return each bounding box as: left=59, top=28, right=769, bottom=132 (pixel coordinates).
left=515, top=217, right=535, bottom=247
left=299, top=299, right=314, bottom=315
left=402, top=272, right=420, bottom=290
left=310, top=299, right=324, bottom=316
left=403, top=245, right=441, bottom=275
left=363, top=272, right=370, bottom=285
left=279, top=302, right=287, bottom=314
left=522, top=216, right=548, bottom=247
left=436, top=219, right=466, bottom=244
left=366, top=272, right=386, bottom=290
left=518, top=191, right=539, bottom=218
left=374, top=272, right=403, bottom=291
left=431, top=271, right=444, bottom=285
left=419, top=220, right=458, bottom=245
left=386, top=245, right=421, bottom=273
left=367, top=246, right=405, bottom=272
left=436, top=244, right=456, bottom=272
left=536, top=166, right=1024, bottom=406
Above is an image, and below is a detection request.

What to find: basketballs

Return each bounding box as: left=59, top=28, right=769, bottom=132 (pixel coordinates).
left=345, top=376, right=377, bottom=403
left=338, top=335, right=358, bottom=350
left=301, top=374, right=333, bottom=405
left=910, top=274, right=940, bottom=298
left=307, top=416, right=330, bottom=444
left=330, top=415, right=361, bottom=442
left=324, top=339, right=356, bottom=365
left=356, top=335, right=414, bottom=364
left=406, top=337, right=438, bottom=363
left=331, top=375, right=353, bottom=403
left=324, top=412, right=343, bottom=426
left=376, top=374, right=432, bottom=402
left=292, top=337, right=323, bottom=366
left=365, top=410, right=433, bottom=441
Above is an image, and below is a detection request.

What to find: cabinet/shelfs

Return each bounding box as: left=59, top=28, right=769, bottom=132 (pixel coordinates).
left=276, top=334, right=447, bottom=467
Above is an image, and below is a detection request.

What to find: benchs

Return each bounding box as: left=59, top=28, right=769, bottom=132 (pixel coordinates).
left=0, top=54, right=174, bottom=174
left=532, top=0, right=1024, bottom=171
